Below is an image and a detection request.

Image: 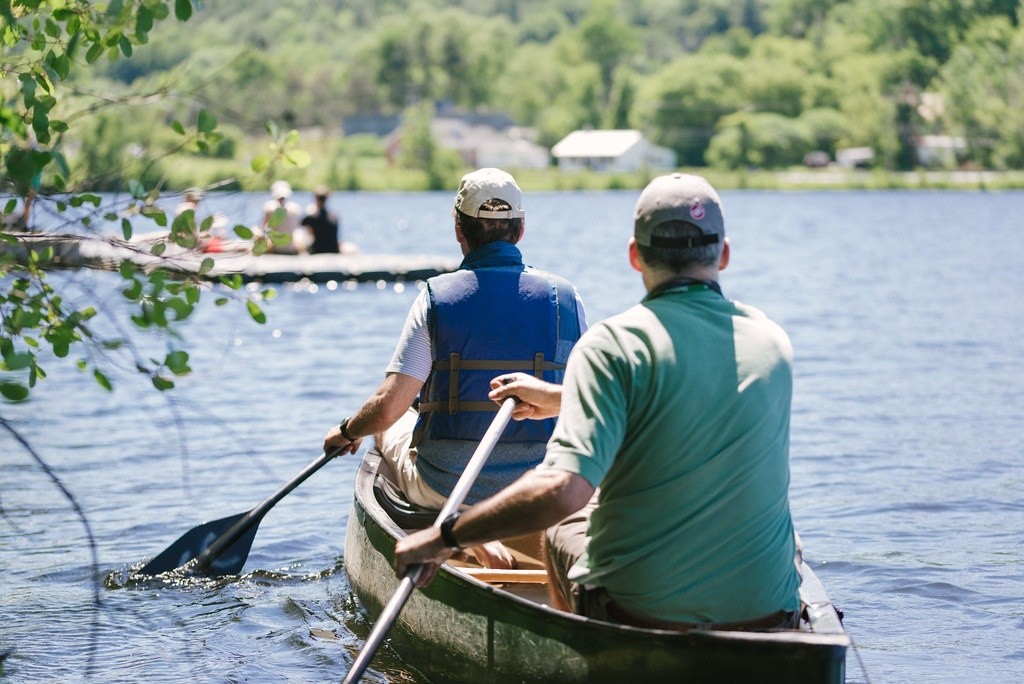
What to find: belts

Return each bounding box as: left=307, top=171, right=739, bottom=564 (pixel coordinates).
left=606, top=605, right=794, bottom=632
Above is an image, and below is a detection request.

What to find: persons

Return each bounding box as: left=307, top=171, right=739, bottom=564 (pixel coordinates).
left=176, top=188, right=249, bottom=252
left=252, top=182, right=340, bottom=255
left=324, top=168, right=589, bottom=512
left=393, top=174, right=802, bottom=632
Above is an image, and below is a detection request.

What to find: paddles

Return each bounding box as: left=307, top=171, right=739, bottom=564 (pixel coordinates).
left=128, top=437, right=361, bottom=582
left=336, top=383, right=520, bottom=684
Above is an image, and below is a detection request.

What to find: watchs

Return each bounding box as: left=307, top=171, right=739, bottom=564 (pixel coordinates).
left=340, top=417, right=359, bottom=442
left=440, top=514, right=467, bottom=552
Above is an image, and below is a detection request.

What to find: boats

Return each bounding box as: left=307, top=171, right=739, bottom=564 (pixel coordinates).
left=343, top=394, right=852, bottom=684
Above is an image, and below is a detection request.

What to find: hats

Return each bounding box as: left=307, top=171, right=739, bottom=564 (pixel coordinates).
left=634, top=172, right=725, bottom=248
left=456, top=167, right=526, bottom=219
